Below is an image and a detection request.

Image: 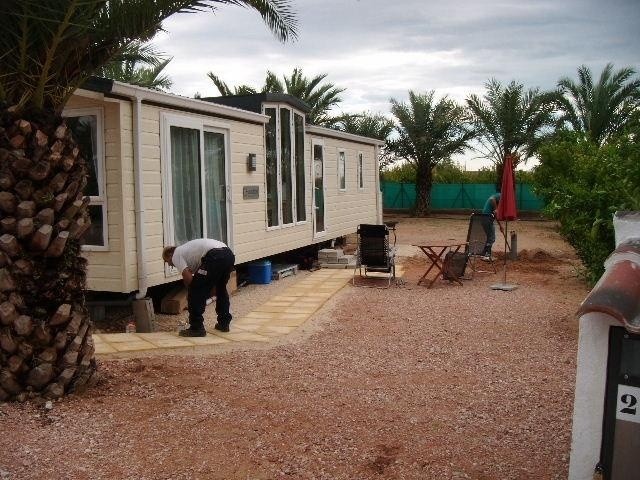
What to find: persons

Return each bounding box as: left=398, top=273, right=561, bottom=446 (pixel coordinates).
left=480, top=193, right=502, bottom=260
left=162, top=236, right=234, bottom=337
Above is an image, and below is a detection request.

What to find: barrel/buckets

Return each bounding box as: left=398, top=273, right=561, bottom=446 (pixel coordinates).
left=249, top=259, right=271, bottom=285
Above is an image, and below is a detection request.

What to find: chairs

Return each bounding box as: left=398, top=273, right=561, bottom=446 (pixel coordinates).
left=353, top=223, right=395, bottom=288
left=443, top=211, right=494, bottom=281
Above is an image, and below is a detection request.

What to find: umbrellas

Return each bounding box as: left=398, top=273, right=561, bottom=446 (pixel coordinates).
left=495, top=153, right=519, bottom=261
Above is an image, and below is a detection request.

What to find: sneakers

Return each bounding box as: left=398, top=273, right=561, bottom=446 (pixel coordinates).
left=215, top=322, right=229, bottom=332
left=480, top=254, right=498, bottom=262
left=179, top=325, right=207, bottom=337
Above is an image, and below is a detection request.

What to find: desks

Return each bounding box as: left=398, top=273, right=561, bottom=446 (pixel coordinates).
left=411, top=240, right=469, bottom=289
